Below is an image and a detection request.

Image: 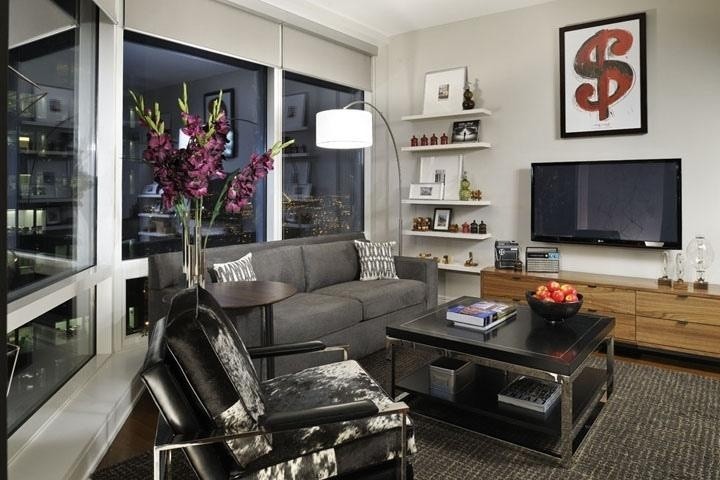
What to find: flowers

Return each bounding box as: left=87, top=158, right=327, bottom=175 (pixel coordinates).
left=130, top=83, right=296, bottom=242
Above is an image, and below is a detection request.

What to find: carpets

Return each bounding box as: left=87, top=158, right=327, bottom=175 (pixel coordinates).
left=90, top=342, right=719, bottom=479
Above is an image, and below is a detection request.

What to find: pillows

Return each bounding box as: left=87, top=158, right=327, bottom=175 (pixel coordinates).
left=353, top=239, right=397, bottom=281
left=212, top=251, right=258, bottom=284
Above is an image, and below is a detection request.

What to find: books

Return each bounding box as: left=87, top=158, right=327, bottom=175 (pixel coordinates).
left=496, top=374, right=563, bottom=415
left=445, top=300, right=518, bottom=335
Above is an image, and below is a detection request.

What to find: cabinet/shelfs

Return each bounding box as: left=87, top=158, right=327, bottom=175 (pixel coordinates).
left=282, top=126, right=315, bottom=229
left=19, top=97, right=79, bottom=263
left=481, top=273, right=720, bottom=361
left=400, top=108, right=490, bottom=298
left=138, top=171, right=227, bottom=251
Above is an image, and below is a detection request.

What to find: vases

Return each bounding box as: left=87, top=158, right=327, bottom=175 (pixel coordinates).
left=179, top=240, right=209, bottom=295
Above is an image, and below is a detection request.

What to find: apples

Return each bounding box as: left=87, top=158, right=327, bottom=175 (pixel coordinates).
left=533, top=280, right=578, bottom=303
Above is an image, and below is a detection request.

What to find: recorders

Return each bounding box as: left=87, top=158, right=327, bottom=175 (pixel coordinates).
left=526, top=247, right=559, bottom=272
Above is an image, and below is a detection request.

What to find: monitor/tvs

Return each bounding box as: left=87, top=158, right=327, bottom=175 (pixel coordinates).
left=531, top=159, right=682, bottom=250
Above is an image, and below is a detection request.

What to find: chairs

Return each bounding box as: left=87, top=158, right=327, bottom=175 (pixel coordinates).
left=139, top=284, right=416, bottom=480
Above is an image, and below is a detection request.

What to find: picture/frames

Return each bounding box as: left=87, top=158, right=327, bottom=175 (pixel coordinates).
left=432, top=208, right=453, bottom=231
left=32, top=82, right=75, bottom=121
left=282, top=92, right=307, bottom=126
left=422, top=66, right=467, bottom=112
left=204, top=90, right=236, bottom=158
left=558, top=12, right=648, bottom=139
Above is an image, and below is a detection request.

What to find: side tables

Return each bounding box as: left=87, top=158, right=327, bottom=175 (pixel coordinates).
left=204, top=280, right=296, bottom=380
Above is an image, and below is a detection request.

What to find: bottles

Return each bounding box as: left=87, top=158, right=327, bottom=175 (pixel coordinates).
left=410, top=133, right=449, bottom=147
left=461, top=220, right=487, bottom=235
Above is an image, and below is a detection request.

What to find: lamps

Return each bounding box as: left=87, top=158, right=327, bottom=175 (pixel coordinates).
left=315, top=98, right=402, bottom=255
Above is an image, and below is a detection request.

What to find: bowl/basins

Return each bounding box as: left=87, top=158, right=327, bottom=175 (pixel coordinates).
left=526, top=289, right=585, bottom=324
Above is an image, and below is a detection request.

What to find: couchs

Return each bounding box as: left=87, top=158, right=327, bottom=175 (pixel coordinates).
left=147, top=232, right=437, bottom=385
